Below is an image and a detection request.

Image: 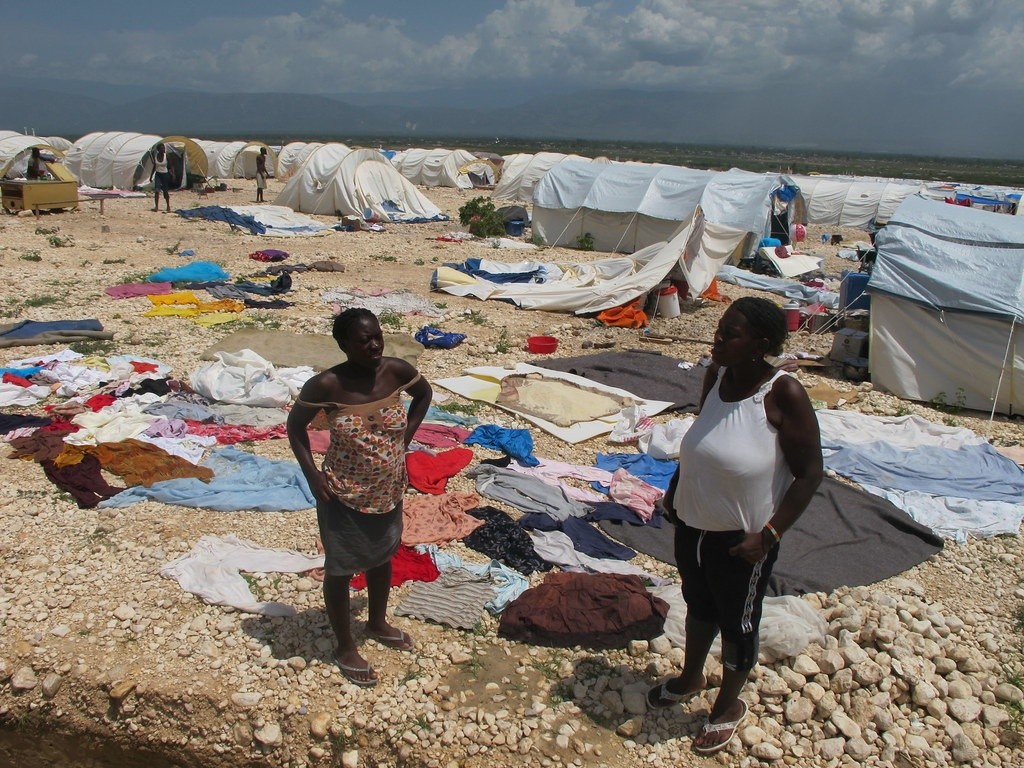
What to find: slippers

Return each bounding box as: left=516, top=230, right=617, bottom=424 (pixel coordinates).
left=646, top=672, right=707, bottom=709
left=365, top=622, right=415, bottom=650
left=694, top=697, right=748, bottom=752
left=334, top=646, right=379, bottom=685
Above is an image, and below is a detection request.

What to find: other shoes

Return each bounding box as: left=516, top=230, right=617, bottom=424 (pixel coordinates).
left=167, top=207, right=170, bottom=211
left=151, top=208, right=158, bottom=211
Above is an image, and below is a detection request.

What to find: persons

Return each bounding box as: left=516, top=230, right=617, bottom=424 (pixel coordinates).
left=150, top=144, right=174, bottom=210
left=646, top=296, right=823, bottom=752
left=287, top=307, right=432, bottom=685
left=257, top=147, right=270, bottom=202
left=27, top=147, right=41, bottom=179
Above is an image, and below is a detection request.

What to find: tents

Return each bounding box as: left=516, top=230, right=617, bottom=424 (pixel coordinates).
left=492, top=151, right=1024, bottom=266
left=274, top=141, right=450, bottom=222
left=65, top=132, right=209, bottom=190
left=866, top=194, right=1024, bottom=416
left=0, top=130, right=73, bottom=178
left=436, top=204, right=757, bottom=317
left=190, top=137, right=506, bottom=190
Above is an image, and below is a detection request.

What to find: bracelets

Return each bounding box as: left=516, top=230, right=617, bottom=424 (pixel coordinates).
left=761, top=523, right=781, bottom=554
